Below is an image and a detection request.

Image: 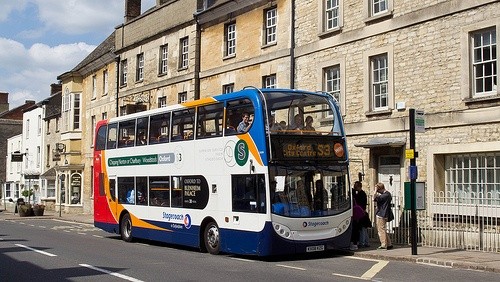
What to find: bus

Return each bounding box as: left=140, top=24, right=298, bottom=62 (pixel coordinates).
left=91, top=86, right=355, bottom=257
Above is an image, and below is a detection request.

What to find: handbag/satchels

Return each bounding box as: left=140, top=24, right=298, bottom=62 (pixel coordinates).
left=359, top=212, right=371, bottom=228
left=388, top=208, right=394, bottom=221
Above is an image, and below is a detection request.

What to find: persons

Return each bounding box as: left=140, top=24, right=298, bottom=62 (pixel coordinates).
left=229, top=108, right=251, bottom=133
left=330, top=188, right=345, bottom=209
left=126, top=189, right=146, bottom=204
left=138, top=131, right=145, bottom=144
left=266, top=114, right=315, bottom=135
left=297, top=180, right=312, bottom=209
left=271, top=180, right=282, bottom=204
left=314, top=180, right=328, bottom=210
left=347, top=181, right=369, bottom=250
left=371, top=183, right=394, bottom=251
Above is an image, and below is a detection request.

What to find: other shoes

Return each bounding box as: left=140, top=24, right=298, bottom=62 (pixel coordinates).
left=365, top=243, right=369, bottom=247
left=358, top=243, right=366, bottom=248
left=387, top=245, right=393, bottom=250
left=376, top=245, right=387, bottom=250
left=350, top=244, right=358, bottom=250
